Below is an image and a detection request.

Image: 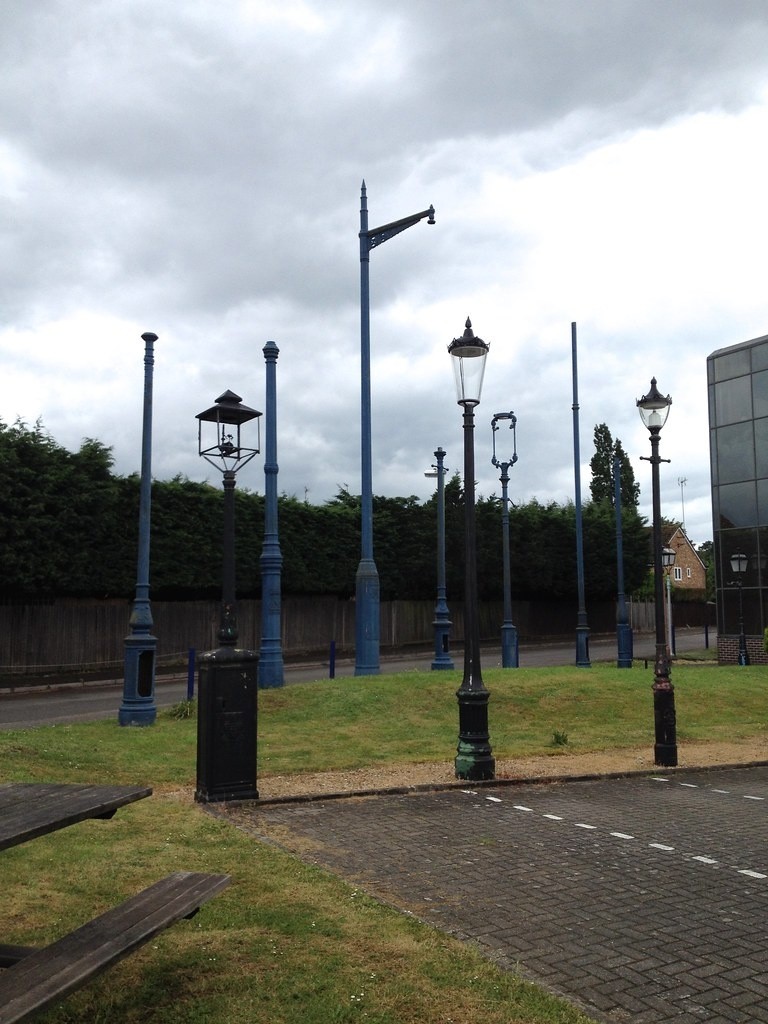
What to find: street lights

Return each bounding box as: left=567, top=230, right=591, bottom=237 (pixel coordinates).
left=634, top=376, right=679, bottom=768
left=191, top=388, right=261, bottom=805
left=447, top=315, right=497, bottom=782
left=659, top=542, right=676, bottom=660
left=728, top=546, right=751, bottom=666
left=423, top=446, right=455, bottom=669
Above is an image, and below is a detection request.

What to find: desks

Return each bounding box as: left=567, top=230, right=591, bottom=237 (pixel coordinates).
left=0, top=782, right=153, bottom=853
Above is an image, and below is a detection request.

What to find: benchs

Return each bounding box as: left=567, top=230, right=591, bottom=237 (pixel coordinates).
left=0, top=871, right=231, bottom=1024
